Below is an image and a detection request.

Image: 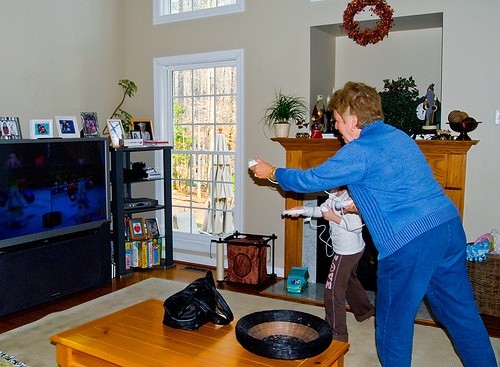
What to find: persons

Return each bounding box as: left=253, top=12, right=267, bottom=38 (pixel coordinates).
left=137, top=122, right=150, bottom=140
left=281, top=184, right=375, bottom=342
left=62, top=120, right=70, bottom=130
left=250, top=82, right=500, bottom=366
left=38, top=123, right=46, bottom=134
left=2, top=121, right=9, bottom=133
left=134, top=132, right=138, bottom=138
left=9, top=125, right=15, bottom=134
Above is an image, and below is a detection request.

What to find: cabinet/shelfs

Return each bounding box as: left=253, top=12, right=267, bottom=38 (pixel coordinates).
left=312, top=94, right=325, bottom=128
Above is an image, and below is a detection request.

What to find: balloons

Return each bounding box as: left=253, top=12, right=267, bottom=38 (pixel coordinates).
left=413, top=97, right=428, bottom=122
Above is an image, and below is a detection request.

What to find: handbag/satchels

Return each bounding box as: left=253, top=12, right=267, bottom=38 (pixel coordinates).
left=269, top=166, right=277, bottom=181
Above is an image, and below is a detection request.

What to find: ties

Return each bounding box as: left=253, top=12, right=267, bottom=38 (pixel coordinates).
left=1, top=137, right=110, bottom=252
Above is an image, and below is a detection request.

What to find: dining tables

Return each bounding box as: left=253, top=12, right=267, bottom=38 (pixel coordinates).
left=102, top=79, right=137, bottom=144
left=257, top=86, right=311, bottom=139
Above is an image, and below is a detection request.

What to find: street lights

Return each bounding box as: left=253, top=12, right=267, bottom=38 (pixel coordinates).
left=163, top=271, right=233, bottom=331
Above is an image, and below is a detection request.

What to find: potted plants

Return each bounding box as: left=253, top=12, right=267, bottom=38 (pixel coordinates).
left=127, top=217, right=147, bottom=240
left=54, top=115, right=81, bottom=138
left=29, top=119, right=54, bottom=139
left=105, top=118, right=127, bottom=149
left=0, top=117, right=23, bottom=140
left=146, top=218, right=160, bottom=237
left=79, top=110, right=100, bottom=138
left=129, top=119, right=155, bottom=143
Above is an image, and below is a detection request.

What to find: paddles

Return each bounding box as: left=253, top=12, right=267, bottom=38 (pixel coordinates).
left=0, top=276, right=500, bottom=366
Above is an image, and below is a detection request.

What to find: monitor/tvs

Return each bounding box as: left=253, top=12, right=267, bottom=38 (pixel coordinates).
left=126, top=236, right=162, bottom=268
left=125, top=237, right=166, bottom=270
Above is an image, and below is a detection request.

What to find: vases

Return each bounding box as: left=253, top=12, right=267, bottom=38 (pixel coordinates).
left=339, top=1, right=394, bottom=46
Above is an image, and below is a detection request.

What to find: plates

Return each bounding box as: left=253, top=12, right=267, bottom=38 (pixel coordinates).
left=109, top=143, right=177, bottom=279
left=1, top=221, right=112, bottom=317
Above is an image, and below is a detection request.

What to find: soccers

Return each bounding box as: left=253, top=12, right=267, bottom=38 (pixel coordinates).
left=461, top=116, right=477, bottom=124
left=460, top=112, right=469, bottom=119
left=451, top=116, right=461, bottom=124
left=448, top=111, right=460, bottom=122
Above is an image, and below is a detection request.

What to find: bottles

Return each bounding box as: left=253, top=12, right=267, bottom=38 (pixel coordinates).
left=138, top=123, right=147, bottom=126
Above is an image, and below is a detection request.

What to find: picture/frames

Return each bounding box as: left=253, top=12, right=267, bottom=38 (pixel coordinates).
left=271, top=137, right=480, bottom=281
